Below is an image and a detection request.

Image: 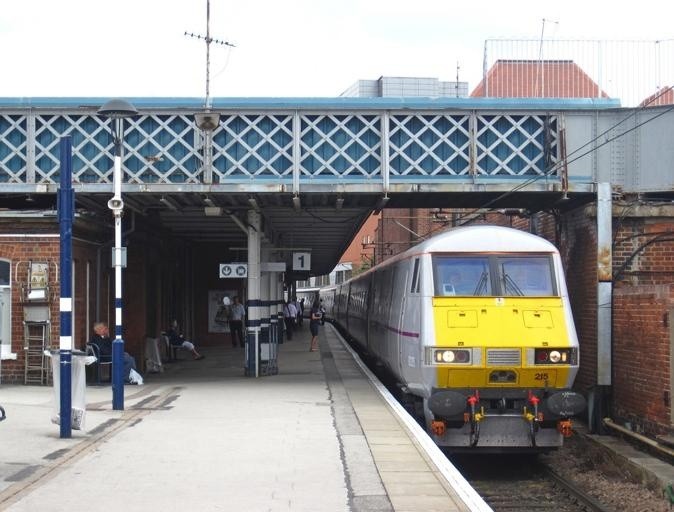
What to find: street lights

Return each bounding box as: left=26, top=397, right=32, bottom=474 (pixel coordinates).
left=92, top=93, right=144, bottom=412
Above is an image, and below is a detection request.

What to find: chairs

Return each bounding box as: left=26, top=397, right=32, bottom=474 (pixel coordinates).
left=164, top=333, right=186, bottom=361
left=88, top=342, right=112, bottom=384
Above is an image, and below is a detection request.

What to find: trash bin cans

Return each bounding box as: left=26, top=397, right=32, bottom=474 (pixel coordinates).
left=43, top=348, right=98, bottom=431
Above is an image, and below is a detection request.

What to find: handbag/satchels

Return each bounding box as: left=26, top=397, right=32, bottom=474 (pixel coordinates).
left=291, top=316, right=298, bottom=329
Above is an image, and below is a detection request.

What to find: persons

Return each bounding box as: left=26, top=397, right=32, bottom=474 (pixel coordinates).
left=226, top=296, right=245, bottom=349
left=309, top=299, right=326, bottom=352
left=92, top=324, right=138, bottom=385
left=168, top=318, right=203, bottom=360
left=283, top=296, right=305, bottom=340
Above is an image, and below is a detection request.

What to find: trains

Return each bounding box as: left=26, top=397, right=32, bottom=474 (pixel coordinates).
left=283, top=219, right=590, bottom=465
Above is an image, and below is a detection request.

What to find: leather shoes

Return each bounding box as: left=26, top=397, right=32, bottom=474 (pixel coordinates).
left=233, top=343, right=245, bottom=347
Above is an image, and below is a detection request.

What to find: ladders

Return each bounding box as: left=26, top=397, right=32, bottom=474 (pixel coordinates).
left=24, top=321, right=50, bottom=385
